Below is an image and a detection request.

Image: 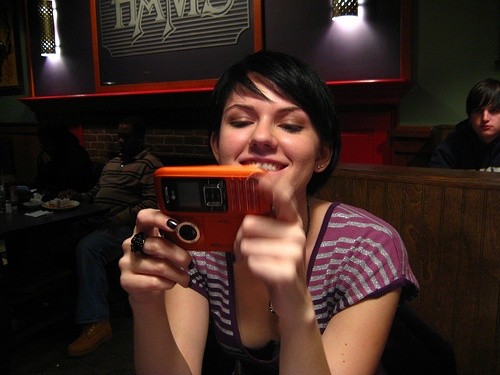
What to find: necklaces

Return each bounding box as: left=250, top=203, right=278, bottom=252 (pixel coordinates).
left=269, top=194, right=311, bottom=314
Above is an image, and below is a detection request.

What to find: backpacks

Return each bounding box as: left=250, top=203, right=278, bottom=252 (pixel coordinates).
left=381, top=305, right=458, bottom=375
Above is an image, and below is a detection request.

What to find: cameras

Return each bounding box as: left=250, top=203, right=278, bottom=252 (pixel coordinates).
left=153, top=166, right=274, bottom=252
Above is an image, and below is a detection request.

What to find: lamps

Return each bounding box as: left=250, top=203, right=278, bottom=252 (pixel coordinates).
left=331, top=0, right=357, bottom=17
left=31, top=0, right=55, bottom=56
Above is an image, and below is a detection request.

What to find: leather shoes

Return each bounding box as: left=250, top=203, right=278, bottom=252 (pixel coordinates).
left=67, top=321, right=112, bottom=355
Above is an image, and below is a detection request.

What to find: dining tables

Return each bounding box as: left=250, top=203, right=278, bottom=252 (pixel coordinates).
left=0, top=197, right=111, bottom=375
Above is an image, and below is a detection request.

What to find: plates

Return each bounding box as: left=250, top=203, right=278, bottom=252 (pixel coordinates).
left=42, top=200, right=80, bottom=210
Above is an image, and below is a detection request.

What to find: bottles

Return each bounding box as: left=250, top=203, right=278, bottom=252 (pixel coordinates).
left=6, top=200, right=12, bottom=214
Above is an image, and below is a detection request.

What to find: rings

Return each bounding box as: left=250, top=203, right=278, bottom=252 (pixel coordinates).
left=130, top=231, right=148, bottom=256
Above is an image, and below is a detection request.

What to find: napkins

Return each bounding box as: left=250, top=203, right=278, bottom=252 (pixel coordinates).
left=24, top=209, right=54, bottom=218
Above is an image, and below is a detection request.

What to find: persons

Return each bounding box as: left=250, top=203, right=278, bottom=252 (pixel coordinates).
left=35, top=115, right=164, bottom=356
left=4, top=116, right=95, bottom=271
left=431, top=78, right=500, bottom=168
left=118, top=49, right=422, bottom=375
